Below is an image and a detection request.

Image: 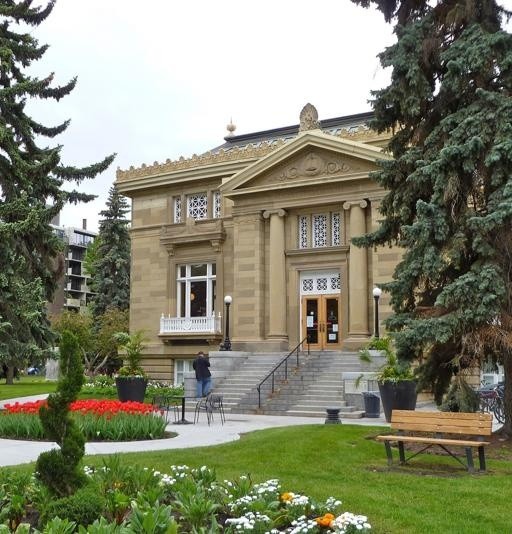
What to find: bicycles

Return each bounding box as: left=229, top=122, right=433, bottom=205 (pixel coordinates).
left=478, top=385, right=506, bottom=424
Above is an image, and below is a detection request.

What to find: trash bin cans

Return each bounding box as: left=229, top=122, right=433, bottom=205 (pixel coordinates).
left=362, top=392, right=381, bottom=418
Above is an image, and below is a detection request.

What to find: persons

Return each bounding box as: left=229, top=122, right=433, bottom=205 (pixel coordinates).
left=495, top=378, right=505, bottom=423
left=193, top=351, right=211, bottom=401
left=83, top=366, right=119, bottom=379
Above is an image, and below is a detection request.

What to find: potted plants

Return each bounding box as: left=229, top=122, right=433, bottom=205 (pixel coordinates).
left=354, top=327, right=421, bottom=423
left=112, top=325, right=152, bottom=404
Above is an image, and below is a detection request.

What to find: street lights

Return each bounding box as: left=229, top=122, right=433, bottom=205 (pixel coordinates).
left=371, top=287, right=383, bottom=338
left=218, top=294, right=234, bottom=351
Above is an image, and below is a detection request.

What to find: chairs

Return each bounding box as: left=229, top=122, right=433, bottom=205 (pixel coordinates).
left=151, top=395, right=226, bottom=427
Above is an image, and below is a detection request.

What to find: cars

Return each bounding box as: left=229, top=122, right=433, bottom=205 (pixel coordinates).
left=24, top=367, right=40, bottom=375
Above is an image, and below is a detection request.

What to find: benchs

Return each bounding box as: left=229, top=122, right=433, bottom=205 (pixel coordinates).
left=377, top=409, right=493, bottom=474
